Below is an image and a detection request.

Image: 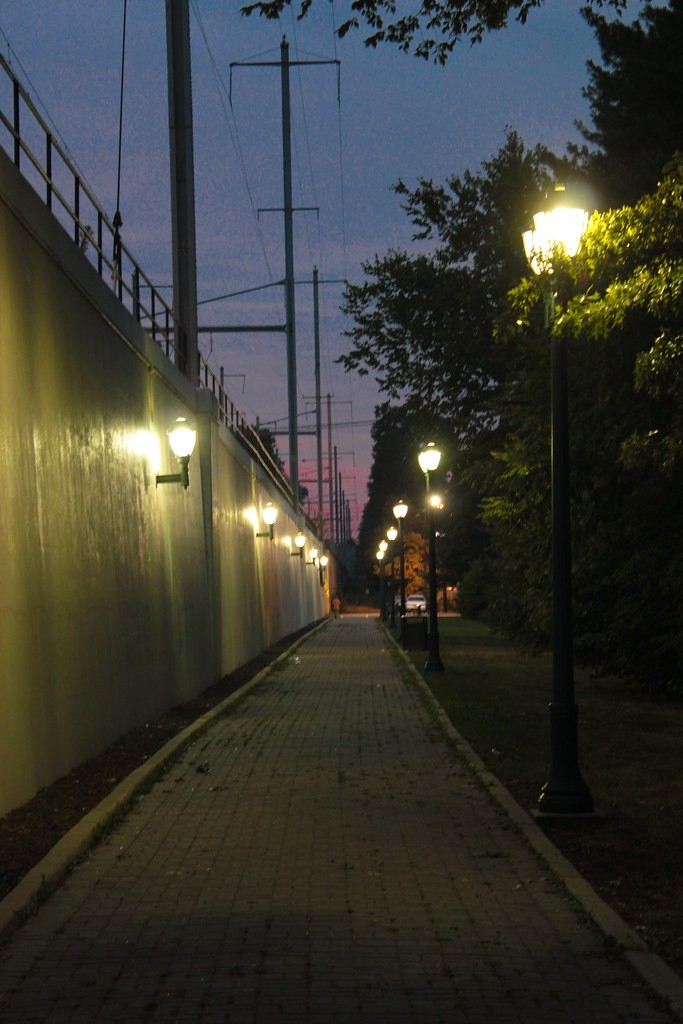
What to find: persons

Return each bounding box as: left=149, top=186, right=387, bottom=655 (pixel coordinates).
left=332, top=595, right=341, bottom=618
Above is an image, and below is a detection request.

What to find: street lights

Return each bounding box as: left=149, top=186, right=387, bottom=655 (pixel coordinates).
left=375, top=442, right=448, bottom=672
left=521, top=206, right=608, bottom=831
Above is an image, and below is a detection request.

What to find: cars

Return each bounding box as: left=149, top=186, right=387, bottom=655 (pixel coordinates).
left=405, top=594, right=427, bottom=612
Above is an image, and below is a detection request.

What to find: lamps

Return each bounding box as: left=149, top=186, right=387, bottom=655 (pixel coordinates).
left=305, top=546, right=317, bottom=565
left=290, top=530, right=305, bottom=557
left=156, top=416, right=196, bottom=489
left=256, top=500, right=278, bottom=541
left=318, top=556, right=328, bottom=572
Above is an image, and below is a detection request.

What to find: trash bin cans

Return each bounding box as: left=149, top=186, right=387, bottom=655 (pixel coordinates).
left=403, top=617, right=428, bottom=650
